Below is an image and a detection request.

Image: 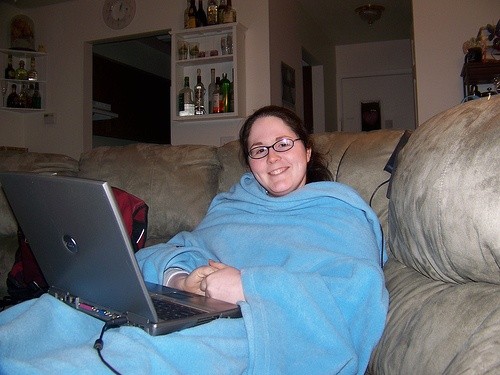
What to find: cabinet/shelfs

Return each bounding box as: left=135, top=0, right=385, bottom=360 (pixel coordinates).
left=168, top=22, right=248, bottom=123
left=0, top=49, right=50, bottom=113
left=460, top=62, right=500, bottom=103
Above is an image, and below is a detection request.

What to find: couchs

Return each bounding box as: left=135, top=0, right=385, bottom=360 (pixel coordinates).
left=0, top=96, right=500, bottom=375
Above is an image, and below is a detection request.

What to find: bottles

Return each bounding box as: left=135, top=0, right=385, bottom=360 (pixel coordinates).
left=5, top=55, right=38, bottom=81
left=7, top=83, right=41, bottom=109
left=178, top=68, right=234, bottom=117
left=183, top=0, right=236, bottom=29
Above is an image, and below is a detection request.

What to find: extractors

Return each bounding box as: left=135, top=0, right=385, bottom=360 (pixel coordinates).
left=92, top=100, right=119, bottom=120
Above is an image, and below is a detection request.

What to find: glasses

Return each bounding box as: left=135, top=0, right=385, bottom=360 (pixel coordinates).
left=245, top=137, right=301, bottom=159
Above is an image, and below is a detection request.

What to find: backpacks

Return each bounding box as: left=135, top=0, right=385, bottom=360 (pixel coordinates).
left=6, top=185, right=150, bottom=306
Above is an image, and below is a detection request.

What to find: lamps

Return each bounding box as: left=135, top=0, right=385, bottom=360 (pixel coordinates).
left=354, top=0, right=385, bottom=25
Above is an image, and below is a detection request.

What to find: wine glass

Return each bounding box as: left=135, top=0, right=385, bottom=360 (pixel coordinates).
left=0, top=81, right=8, bottom=107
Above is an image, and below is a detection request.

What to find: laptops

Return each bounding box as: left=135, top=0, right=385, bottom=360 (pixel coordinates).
left=0, top=170, right=244, bottom=336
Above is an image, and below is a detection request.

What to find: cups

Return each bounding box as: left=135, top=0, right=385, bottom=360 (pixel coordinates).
left=177, top=35, right=232, bottom=60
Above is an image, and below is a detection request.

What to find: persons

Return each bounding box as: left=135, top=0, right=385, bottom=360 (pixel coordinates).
left=0, top=105, right=391, bottom=375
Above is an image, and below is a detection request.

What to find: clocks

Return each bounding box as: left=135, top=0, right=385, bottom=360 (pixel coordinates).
left=100, top=0, right=137, bottom=32
